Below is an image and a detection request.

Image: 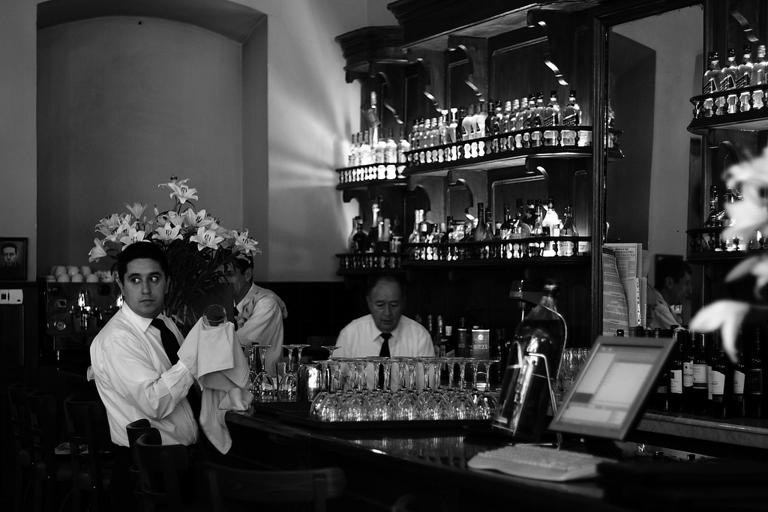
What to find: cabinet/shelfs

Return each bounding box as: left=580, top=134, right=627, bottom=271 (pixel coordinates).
left=386, top=0, right=592, bottom=349
left=43, top=279, right=120, bottom=336
left=329, top=24, right=402, bottom=277
left=688, top=0, right=768, bottom=300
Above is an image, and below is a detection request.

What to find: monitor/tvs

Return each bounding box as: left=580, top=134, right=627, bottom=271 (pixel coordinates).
left=549, top=336, right=677, bottom=453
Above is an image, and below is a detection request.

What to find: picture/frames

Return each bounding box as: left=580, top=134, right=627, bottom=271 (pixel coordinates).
left=0, top=236, right=29, bottom=281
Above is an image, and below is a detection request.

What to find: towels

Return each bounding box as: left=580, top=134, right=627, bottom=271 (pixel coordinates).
left=174, top=316, right=257, bottom=458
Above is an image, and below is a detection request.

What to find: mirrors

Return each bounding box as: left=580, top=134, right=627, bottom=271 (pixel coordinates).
left=599, top=0, right=707, bottom=338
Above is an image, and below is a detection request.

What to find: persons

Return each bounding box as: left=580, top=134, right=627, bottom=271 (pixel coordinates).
left=331, top=271, right=440, bottom=389
left=0, top=243, right=24, bottom=281
left=224, top=246, right=284, bottom=390
left=89, top=242, right=198, bottom=511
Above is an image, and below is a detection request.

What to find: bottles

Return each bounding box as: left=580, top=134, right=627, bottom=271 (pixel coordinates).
left=488, top=282, right=564, bottom=445
left=346, top=203, right=402, bottom=269
left=408, top=198, right=579, bottom=260
left=614, top=325, right=766, bottom=418
left=347, top=125, right=409, bottom=184
left=409, top=98, right=485, bottom=165
left=485, top=88, right=581, bottom=154
left=425, top=311, right=491, bottom=357
left=697, top=44, right=768, bottom=119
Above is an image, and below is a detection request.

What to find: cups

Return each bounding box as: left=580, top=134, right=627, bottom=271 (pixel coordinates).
left=557, top=346, right=592, bottom=384
left=54, top=265, right=99, bottom=282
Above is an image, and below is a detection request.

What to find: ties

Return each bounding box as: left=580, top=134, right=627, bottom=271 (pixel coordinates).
left=149, top=318, right=205, bottom=436
left=231, top=307, right=240, bottom=331
left=378, top=332, right=393, bottom=391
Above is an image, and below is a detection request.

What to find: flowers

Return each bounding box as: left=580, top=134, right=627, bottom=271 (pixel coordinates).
left=89, top=175, right=262, bottom=313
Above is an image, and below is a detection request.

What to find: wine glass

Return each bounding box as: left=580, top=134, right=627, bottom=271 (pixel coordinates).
left=294, top=342, right=311, bottom=363
left=251, top=345, right=274, bottom=404
left=317, top=343, right=343, bottom=357
left=203, top=304, right=227, bottom=327
left=309, top=356, right=496, bottom=420
left=277, top=342, right=297, bottom=403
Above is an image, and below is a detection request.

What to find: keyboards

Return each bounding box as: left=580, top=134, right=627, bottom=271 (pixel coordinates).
left=467, top=444, right=617, bottom=481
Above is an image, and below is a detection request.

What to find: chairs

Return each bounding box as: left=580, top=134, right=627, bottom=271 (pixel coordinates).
left=206, top=462, right=346, bottom=512
left=4, top=381, right=197, bottom=512
left=391, top=492, right=417, bottom=512
